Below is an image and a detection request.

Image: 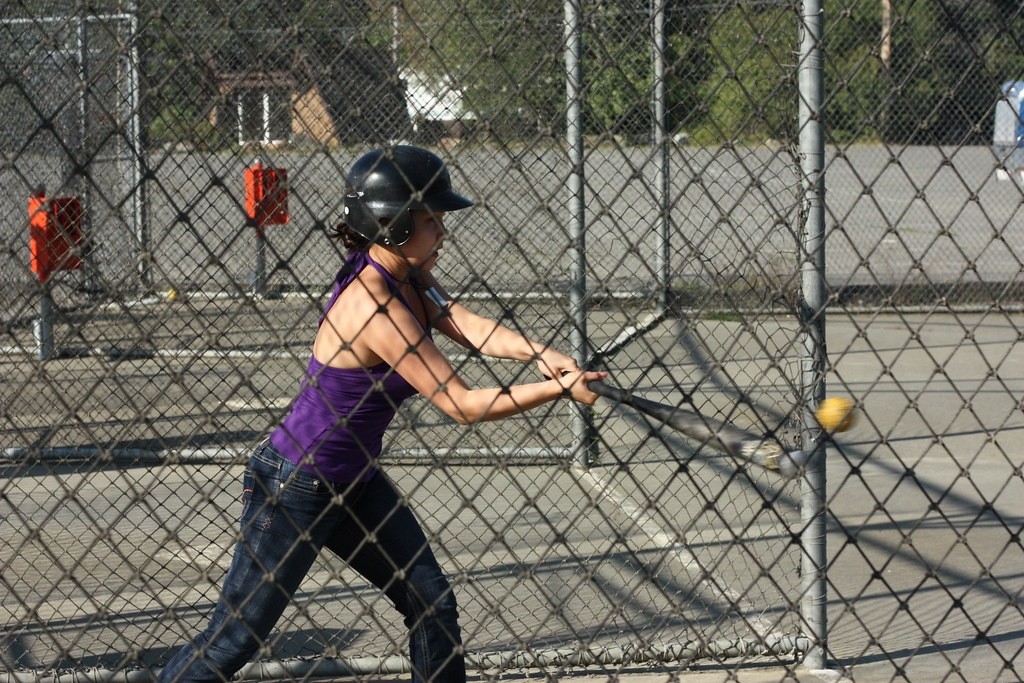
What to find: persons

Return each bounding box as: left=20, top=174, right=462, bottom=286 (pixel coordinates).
left=160, top=145, right=607, bottom=682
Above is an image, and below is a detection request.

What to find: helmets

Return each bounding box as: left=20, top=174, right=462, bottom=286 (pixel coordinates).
left=343, top=146, right=474, bottom=250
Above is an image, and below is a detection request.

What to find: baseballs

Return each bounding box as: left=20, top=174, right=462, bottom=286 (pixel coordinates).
left=816, top=397, right=858, bottom=432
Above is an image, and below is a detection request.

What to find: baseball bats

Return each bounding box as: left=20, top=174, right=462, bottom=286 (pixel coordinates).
left=544, top=370, right=810, bottom=479
